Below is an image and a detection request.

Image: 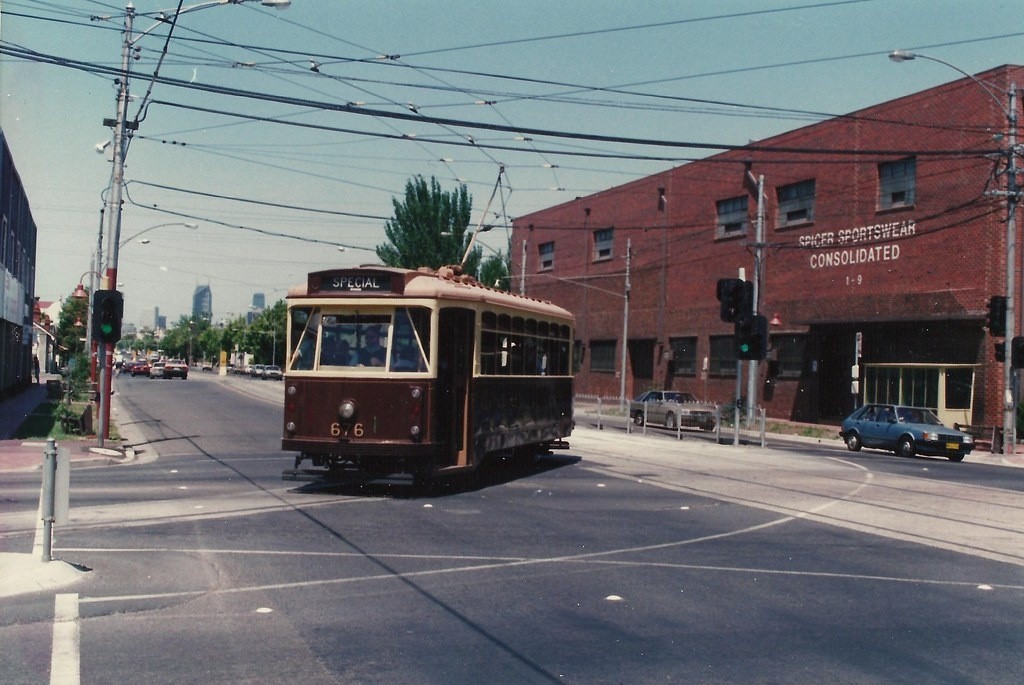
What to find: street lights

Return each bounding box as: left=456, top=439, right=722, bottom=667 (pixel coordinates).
left=439, top=230, right=528, bottom=297
left=88, top=221, right=199, bottom=400
left=889, top=49, right=1019, bottom=454
left=96, top=0, right=293, bottom=440
left=248, top=303, right=277, bottom=367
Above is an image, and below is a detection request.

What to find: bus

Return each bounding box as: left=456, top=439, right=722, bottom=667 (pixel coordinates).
left=279, top=163, right=576, bottom=484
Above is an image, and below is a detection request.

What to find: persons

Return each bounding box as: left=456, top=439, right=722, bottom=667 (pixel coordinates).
left=34, top=356, right=41, bottom=385
left=297, top=321, right=422, bottom=374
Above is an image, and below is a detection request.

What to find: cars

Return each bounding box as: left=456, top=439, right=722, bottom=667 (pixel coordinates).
left=838, top=402, right=976, bottom=462
left=111, top=348, right=284, bottom=381
left=628, top=389, right=716, bottom=431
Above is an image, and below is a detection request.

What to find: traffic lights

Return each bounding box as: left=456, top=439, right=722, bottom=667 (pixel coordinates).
left=993, top=341, right=1006, bottom=363
left=985, top=296, right=1006, bottom=337
left=716, top=277, right=747, bottom=323
left=734, top=313, right=768, bottom=360
left=1010, top=335, right=1024, bottom=370
left=89, top=288, right=123, bottom=343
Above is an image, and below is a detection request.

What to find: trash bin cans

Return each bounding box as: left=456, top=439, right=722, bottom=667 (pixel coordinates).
left=47, top=380, right=60, bottom=398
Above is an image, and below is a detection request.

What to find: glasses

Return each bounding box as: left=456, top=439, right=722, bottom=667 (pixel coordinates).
left=364, top=334, right=375, bottom=338
